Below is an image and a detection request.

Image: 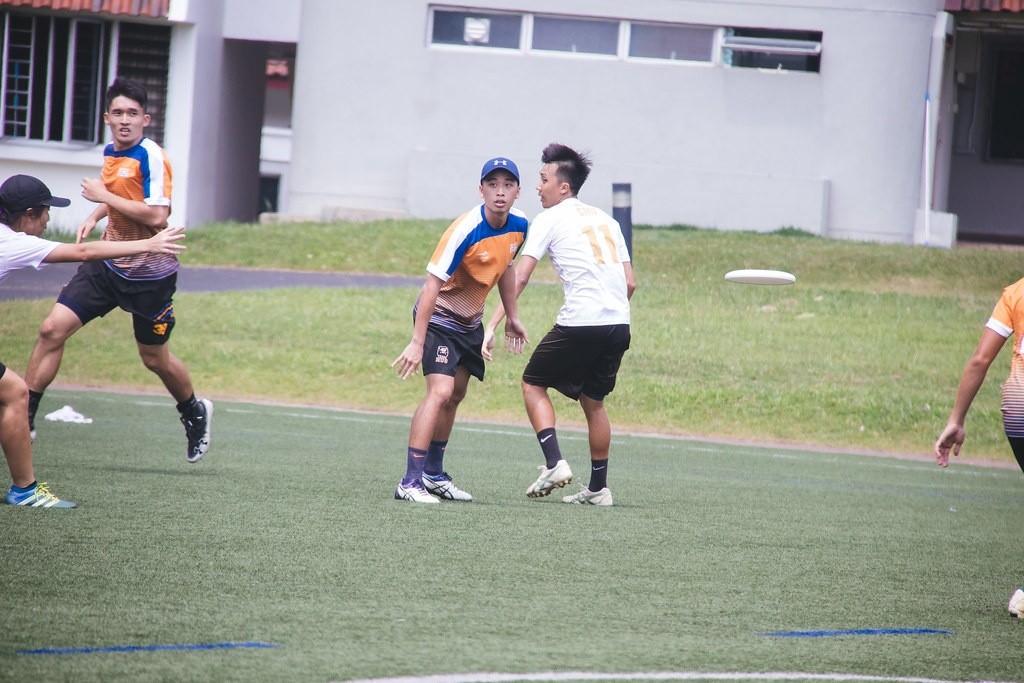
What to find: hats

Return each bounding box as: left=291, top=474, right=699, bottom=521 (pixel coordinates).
left=479, top=158, right=519, bottom=189
left=1, top=174, right=70, bottom=214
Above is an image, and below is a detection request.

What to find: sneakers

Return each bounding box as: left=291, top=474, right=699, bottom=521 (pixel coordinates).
left=562, top=478, right=614, bottom=506
left=180, top=399, right=214, bottom=464
left=525, top=459, right=573, bottom=499
left=4, top=480, right=77, bottom=509
left=394, top=479, right=441, bottom=504
left=420, top=471, right=474, bottom=503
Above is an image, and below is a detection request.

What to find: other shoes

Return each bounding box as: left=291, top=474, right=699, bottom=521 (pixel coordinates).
left=1007, top=586, right=1024, bottom=619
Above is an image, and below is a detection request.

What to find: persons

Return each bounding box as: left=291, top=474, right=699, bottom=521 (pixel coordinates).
left=0, top=174, right=187, bottom=508
left=391, top=157, right=531, bottom=503
left=22, top=78, right=213, bottom=461
left=481, top=142, right=637, bottom=507
left=934, top=269, right=1024, bottom=620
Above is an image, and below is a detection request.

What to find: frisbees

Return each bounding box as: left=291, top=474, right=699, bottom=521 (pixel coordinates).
left=723, top=269, right=796, bottom=285
